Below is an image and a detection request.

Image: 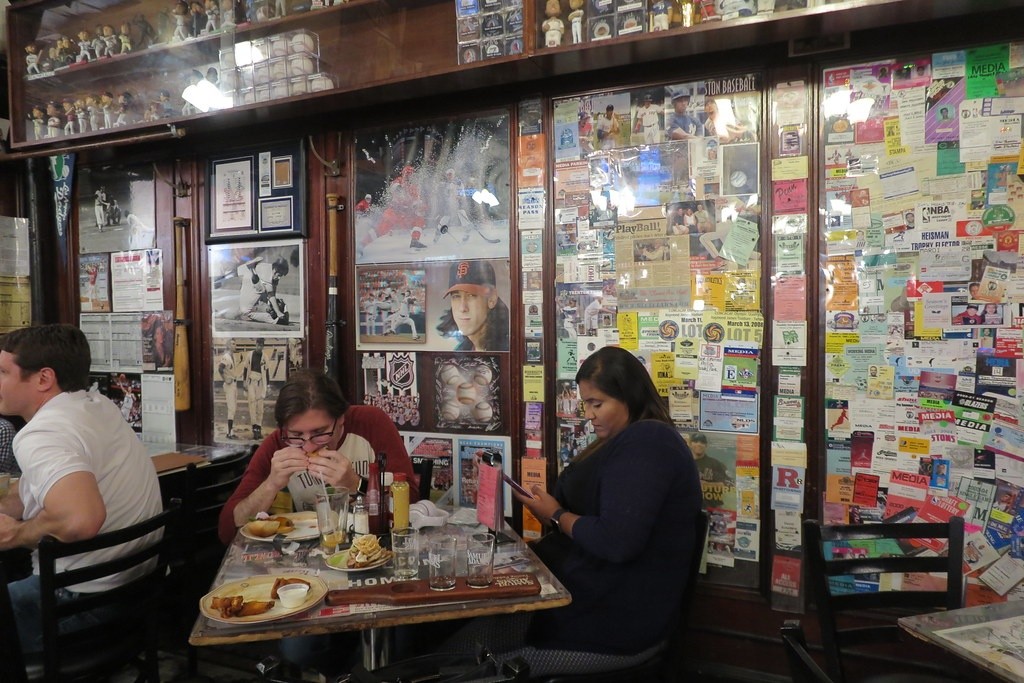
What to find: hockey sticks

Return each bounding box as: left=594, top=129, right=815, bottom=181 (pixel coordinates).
left=426, top=210, right=470, bottom=243
left=457, top=210, right=501, bottom=243
left=236, top=353, right=244, bottom=368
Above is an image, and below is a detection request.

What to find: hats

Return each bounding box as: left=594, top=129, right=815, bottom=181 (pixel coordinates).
left=580, top=113, right=590, bottom=120
left=691, top=433, right=707, bottom=443
left=606, top=104, right=614, bottom=111
left=442, top=261, right=496, bottom=299
left=642, top=95, right=653, bottom=102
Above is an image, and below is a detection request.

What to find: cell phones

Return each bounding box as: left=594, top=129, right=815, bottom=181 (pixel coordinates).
left=501, top=473, right=535, bottom=502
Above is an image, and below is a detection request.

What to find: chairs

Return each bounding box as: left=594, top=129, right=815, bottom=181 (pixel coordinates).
left=543, top=508, right=709, bottom=683
left=24, top=443, right=261, bottom=683
left=777, top=516, right=983, bottom=683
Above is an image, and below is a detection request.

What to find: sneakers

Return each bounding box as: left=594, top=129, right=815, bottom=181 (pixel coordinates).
left=408, top=238, right=428, bottom=254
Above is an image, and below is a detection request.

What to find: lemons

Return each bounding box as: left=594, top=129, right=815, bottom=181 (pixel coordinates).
left=322, top=530, right=345, bottom=543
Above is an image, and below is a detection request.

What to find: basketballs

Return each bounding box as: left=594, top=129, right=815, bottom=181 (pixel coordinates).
left=119, top=374, right=126, bottom=382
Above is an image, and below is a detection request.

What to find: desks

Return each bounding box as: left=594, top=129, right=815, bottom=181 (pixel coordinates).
left=897, top=599, right=1024, bottom=683
left=0, top=445, right=245, bottom=584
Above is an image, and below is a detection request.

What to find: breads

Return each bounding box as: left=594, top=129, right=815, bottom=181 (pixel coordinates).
left=305, top=447, right=331, bottom=460
left=248, top=520, right=280, bottom=537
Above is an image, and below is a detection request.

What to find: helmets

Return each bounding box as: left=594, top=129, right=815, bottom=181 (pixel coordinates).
left=670, top=87, right=691, bottom=106
left=365, top=194, right=372, bottom=203
left=569, top=297, right=577, bottom=305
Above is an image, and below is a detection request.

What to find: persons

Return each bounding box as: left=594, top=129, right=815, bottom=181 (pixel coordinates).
left=905, top=213, right=915, bottom=229
left=85, top=262, right=105, bottom=310
left=118, top=374, right=137, bottom=423
left=952, top=283, right=1003, bottom=337
left=142, top=314, right=173, bottom=368
left=939, top=108, right=953, bottom=122
left=23, top=0, right=285, bottom=140
left=539, top=1, right=674, bottom=48
left=558, top=381, right=589, bottom=468
left=869, top=366, right=877, bottom=377
left=363, top=391, right=420, bottom=427
left=829, top=150, right=842, bottom=165
left=238, top=256, right=290, bottom=325
left=217, top=369, right=420, bottom=667
left=578, top=87, right=757, bottom=271
left=220, top=338, right=270, bottom=441
left=353, top=158, right=510, bottom=350
left=878, top=67, right=925, bottom=78
left=431, top=347, right=702, bottom=683
left=0, top=324, right=164, bottom=655
left=93, top=184, right=155, bottom=250
left=560, top=296, right=617, bottom=339
left=689, top=432, right=736, bottom=513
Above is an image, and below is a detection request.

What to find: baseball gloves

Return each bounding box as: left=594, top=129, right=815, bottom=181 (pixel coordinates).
left=407, top=297, right=416, bottom=304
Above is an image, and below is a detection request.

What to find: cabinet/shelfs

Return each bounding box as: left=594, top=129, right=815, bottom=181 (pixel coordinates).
left=1, top=0, right=1022, bottom=154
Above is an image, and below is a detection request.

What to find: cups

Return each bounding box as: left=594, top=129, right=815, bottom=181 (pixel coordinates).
left=312, top=487, right=349, bottom=554
left=391, top=527, right=420, bottom=580
left=428, top=535, right=458, bottom=591
left=466, top=532, right=495, bottom=589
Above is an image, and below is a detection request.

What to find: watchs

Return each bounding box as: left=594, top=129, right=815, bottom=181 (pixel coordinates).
left=350, top=475, right=368, bottom=499
left=549, top=507, right=571, bottom=535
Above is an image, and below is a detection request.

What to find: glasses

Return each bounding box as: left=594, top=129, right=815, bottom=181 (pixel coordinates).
left=280, top=418, right=337, bottom=447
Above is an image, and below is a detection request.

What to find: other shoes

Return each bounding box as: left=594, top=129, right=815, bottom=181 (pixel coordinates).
left=252, top=424, right=264, bottom=440
left=226, top=431, right=238, bottom=439
left=246, top=257, right=264, bottom=270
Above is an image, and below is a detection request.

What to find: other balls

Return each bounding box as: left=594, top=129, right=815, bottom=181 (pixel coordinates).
left=440, top=225, right=448, bottom=234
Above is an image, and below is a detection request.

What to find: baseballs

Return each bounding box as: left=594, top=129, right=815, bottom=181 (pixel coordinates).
left=441, top=365, right=459, bottom=385
left=457, top=383, right=477, bottom=404
left=442, top=401, right=460, bottom=421
left=475, top=402, right=492, bottom=422
left=474, top=365, right=495, bottom=386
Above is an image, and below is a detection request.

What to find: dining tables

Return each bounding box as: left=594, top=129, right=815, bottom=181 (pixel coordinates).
left=189, top=505, right=571, bottom=672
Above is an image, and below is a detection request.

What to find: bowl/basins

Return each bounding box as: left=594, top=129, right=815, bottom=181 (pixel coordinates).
left=277, top=584, right=309, bottom=609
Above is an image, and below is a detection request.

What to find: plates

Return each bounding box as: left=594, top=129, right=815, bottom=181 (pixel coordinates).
left=325, top=548, right=393, bottom=571
left=199, top=573, right=329, bottom=625
left=240, top=510, right=339, bottom=542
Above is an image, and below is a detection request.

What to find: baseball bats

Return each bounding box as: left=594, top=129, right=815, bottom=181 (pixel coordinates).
left=174, top=217, right=190, bottom=411
left=325, top=193, right=340, bottom=379
left=555, top=297, right=568, bottom=318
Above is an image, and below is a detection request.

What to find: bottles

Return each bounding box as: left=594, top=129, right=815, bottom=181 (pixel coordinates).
left=353, top=497, right=370, bottom=541
left=387, top=473, right=410, bottom=537
